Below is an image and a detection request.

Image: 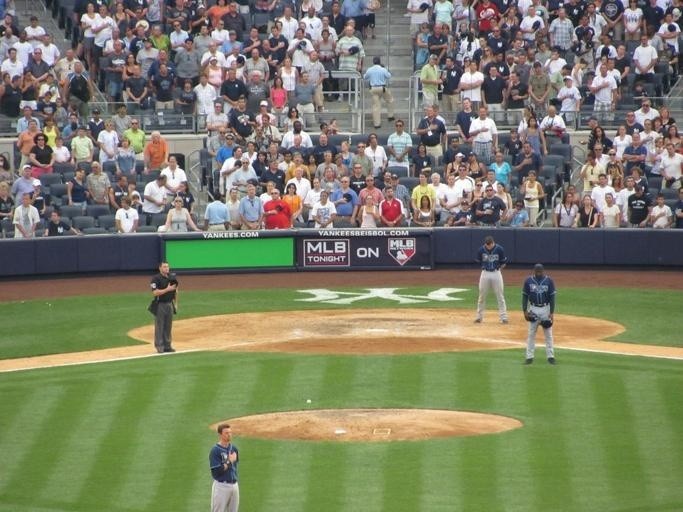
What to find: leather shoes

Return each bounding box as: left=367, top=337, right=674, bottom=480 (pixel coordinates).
left=156, top=346, right=165, bottom=353
left=163, top=347, right=176, bottom=352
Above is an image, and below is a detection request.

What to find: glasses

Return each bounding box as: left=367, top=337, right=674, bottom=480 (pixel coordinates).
left=2, top=5, right=680, bottom=227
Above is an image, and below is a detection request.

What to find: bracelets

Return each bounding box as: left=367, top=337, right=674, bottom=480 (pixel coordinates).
left=224, top=460, right=232, bottom=469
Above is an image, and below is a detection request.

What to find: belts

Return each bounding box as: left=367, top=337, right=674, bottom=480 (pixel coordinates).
left=482, top=267, right=498, bottom=272
left=218, top=478, right=237, bottom=483
left=530, top=302, right=548, bottom=307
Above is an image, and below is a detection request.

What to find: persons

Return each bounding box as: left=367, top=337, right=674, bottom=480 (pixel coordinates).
left=474, top=235, right=509, bottom=324
left=364, top=57, right=395, bottom=128
left=201, top=95, right=681, bottom=232
left=521, top=264, right=557, bottom=365
left=209, top=424, right=240, bottom=511
left=149, top=262, right=178, bottom=354
left=57, top=1, right=379, bottom=130
left=406, top=1, right=682, bottom=130
left=1, top=2, right=203, bottom=238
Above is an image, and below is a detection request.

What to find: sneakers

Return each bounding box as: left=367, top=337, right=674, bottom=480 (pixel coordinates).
left=475, top=317, right=482, bottom=323
left=500, top=316, right=508, bottom=322
left=548, top=357, right=556, bottom=364
left=525, top=357, right=533, bottom=365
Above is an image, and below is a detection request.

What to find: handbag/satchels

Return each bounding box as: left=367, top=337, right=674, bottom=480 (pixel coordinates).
left=148, top=297, right=159, bottom=315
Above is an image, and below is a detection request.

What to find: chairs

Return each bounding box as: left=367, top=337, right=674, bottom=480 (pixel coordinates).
left=0, top=1, right=683, bottom=239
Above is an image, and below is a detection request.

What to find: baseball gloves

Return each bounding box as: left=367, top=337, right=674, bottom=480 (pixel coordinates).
left=526, top=310, right=538, bottom=321
